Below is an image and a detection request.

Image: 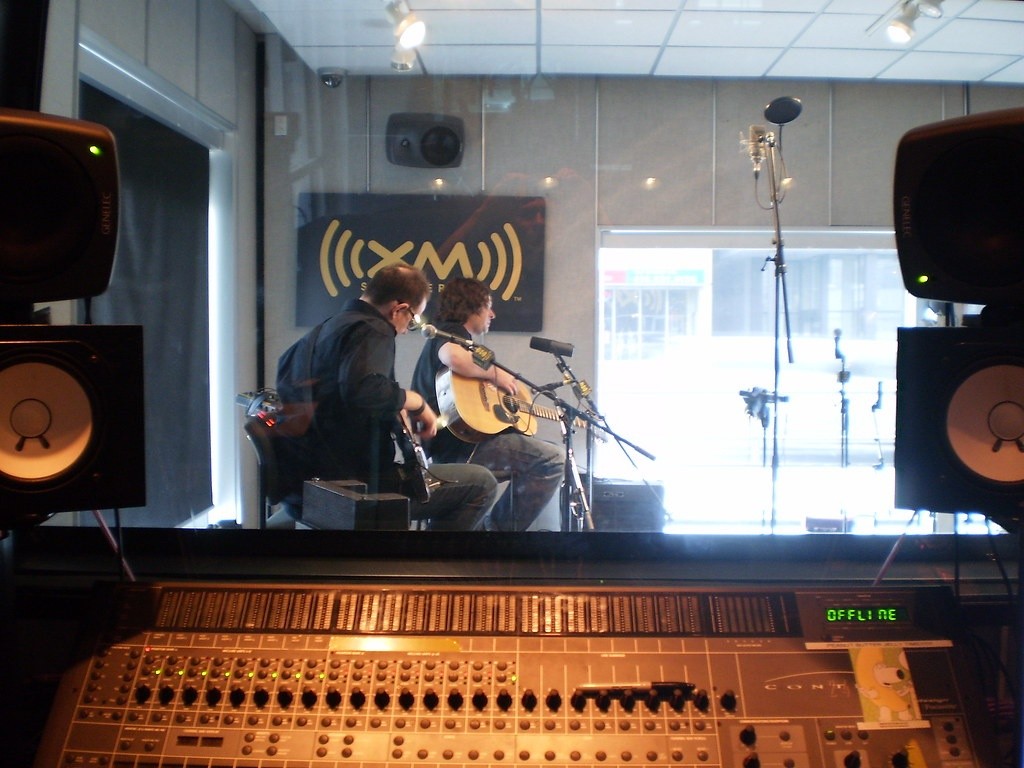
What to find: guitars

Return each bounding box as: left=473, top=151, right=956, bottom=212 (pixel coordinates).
left=435, top=350, right=611, bottom=445
left=393, top=410, right=430, bottom=504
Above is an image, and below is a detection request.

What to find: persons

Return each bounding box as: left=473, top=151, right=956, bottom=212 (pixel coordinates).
left=410, top=277, right=565, bottom=532
left=275, top=262, right=498, bottom=532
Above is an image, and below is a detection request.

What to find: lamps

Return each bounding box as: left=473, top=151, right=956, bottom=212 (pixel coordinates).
left=916, top=0, right=944, bottom=19
left=885, top=2, right=920, bottom=46
left=382, top=0, right=428, bottom=73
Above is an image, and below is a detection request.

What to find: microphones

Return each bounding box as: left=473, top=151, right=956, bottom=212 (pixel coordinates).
left=749, top=124, right=767, bottom=179
left=421, top=324, right=479, bottom=347
left=531, top=381, right=575, bottom=395
left=530, top=336, right=572, bottom=358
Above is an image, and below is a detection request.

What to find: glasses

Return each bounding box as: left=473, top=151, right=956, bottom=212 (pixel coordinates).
left=399, top=301, right=424, bottom=331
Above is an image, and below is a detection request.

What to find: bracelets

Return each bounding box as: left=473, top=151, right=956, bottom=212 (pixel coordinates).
left=409, top=394, right=426, bottom=416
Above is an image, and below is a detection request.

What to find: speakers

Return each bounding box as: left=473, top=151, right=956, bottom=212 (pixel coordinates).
left=0, top=107, right=119, bottom=304
left=385, top=113, right=464, bottom=169
left=894, top=109, right=1024, bottom=308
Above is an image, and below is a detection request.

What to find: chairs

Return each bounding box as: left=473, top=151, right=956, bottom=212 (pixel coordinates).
left=412, top=420, right=517, bottom=533
left=244, top=418, right=299, bottom=528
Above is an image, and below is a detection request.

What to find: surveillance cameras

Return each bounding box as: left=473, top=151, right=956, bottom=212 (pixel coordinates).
left=318, top=67, right=346, bottom=88
